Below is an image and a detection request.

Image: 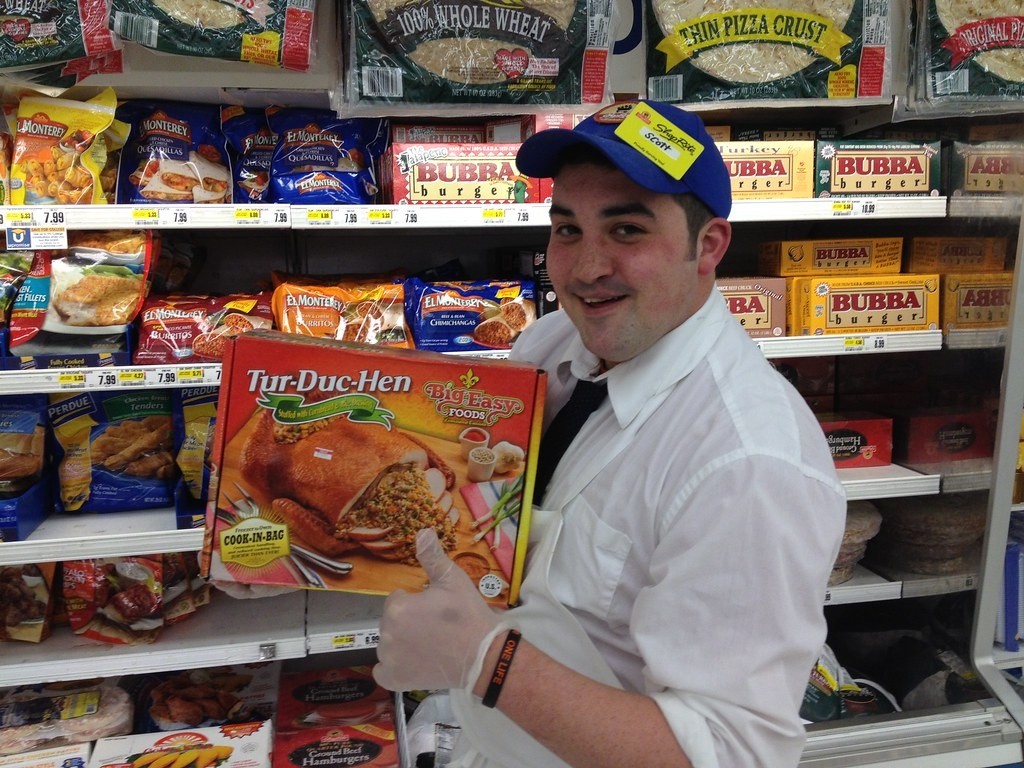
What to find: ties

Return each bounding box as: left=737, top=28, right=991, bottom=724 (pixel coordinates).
left=531, top=379, right=607, bottom=507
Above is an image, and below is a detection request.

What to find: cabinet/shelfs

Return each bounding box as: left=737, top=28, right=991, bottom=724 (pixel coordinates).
left=1, top=0, right=307, bottom=768
left=305, top=1, right=1023, bottom=768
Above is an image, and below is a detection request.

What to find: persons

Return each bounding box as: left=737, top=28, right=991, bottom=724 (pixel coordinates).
left=369, top=98, right=848, bottom=767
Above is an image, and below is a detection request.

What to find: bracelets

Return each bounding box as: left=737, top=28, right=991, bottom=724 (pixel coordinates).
left=480, top=629, right=522, bottom=710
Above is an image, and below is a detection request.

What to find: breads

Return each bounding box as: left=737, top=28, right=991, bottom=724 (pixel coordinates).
left=91, top=415, right=173, bottom=478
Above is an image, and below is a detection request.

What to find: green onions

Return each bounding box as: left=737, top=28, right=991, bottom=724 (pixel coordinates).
left=469, top=471, right=523, bottom=550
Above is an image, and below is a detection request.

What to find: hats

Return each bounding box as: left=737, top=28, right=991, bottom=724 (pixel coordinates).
left=515, top=98, right=732, bottom=220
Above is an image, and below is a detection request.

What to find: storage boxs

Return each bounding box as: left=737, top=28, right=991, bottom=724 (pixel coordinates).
left=388, top=106, right=1023, bottom=474
left=197, top=329, right=548, bottom=610
left=0, top=647, right=404, bottom=768
